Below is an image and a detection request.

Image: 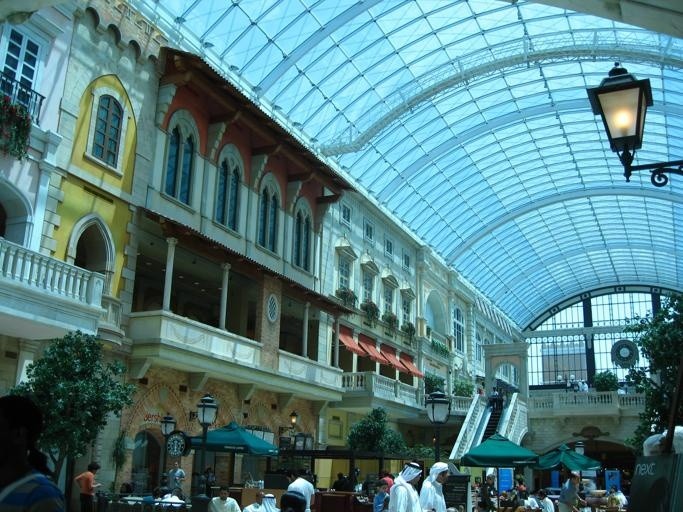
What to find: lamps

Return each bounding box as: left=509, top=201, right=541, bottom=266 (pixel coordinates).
left=278, top=409, right=298, bottom=436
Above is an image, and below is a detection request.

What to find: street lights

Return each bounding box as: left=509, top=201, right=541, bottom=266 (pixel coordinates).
left=425, top=389, right=451, bottom=462
left=556, top=374, right=575, bottom=404
left=574, top=441, right=586, bottom=508
left=191, top=392, right=217, bottom=511
left=155, top=411, right=176, bottom=497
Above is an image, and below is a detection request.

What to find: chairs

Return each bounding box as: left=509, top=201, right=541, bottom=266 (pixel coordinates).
left=112, top=474, right=625, bottom=511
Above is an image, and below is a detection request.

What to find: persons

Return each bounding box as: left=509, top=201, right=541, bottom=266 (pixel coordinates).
left=490, top=386, right=507, bottom=412
left=73, top=462, right=101, bottom=512
left=117, top=460, right=629, bottom=511
left=1, top=394, right=66, bottom=512
left=581, top=381, right=589, bottom=402
